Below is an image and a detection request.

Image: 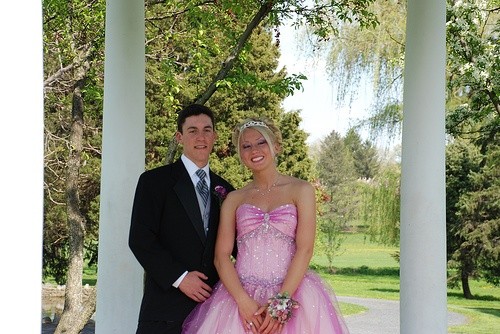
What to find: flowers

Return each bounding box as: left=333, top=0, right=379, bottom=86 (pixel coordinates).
left=209, top=185, right=228, bottom=207
left=267, top=290, right=302, bottom=326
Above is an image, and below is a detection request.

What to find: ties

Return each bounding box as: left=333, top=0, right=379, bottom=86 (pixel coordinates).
left=195, top=168, right=210, bottom=209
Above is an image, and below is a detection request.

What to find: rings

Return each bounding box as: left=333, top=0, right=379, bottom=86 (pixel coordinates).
left=249, top=322, right=253, bottom=326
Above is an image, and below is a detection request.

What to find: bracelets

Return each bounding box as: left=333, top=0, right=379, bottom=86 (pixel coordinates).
left=267, top=292, right=300, bottom=325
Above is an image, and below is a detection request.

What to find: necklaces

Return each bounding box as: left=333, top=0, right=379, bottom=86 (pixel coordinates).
left=253, top=173, right=281, bottom=196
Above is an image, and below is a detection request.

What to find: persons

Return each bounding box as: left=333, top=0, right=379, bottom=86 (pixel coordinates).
left=181, top=118, right=347, bottom=334
left=129, top=104, right=237, bottom=334
